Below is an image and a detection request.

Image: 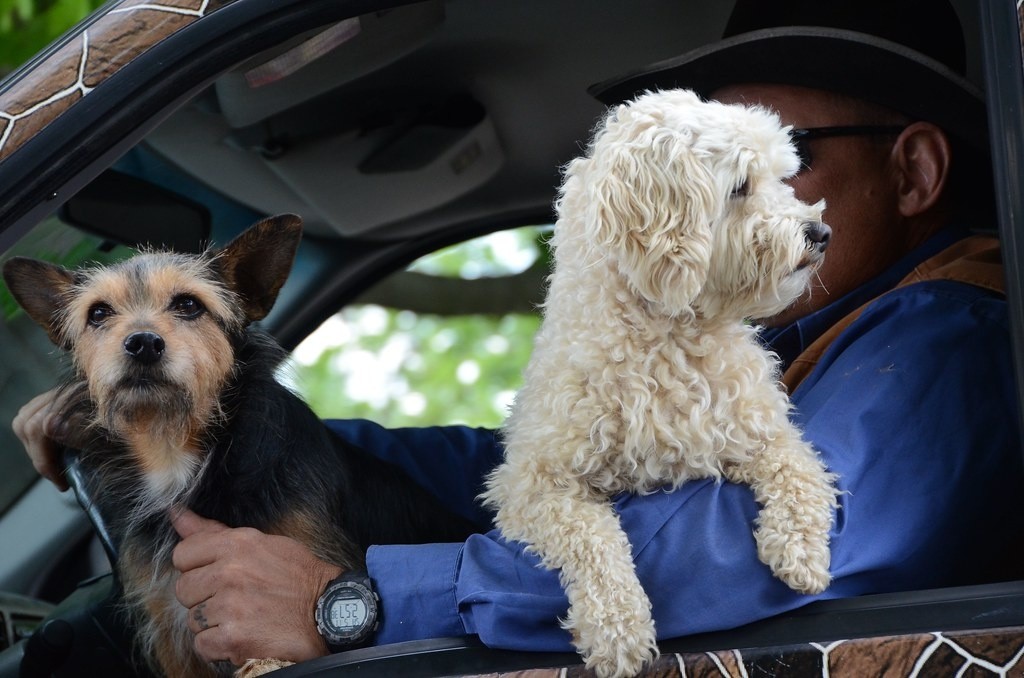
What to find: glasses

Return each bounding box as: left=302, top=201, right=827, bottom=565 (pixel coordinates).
left=790, top=123, right=903, bottom=174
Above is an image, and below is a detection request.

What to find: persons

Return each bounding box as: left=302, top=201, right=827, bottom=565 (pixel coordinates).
left=14, top=2, right=1024, bottom=666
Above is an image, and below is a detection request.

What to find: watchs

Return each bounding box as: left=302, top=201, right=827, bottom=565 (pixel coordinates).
left=314, top=568, right=382, bottom=649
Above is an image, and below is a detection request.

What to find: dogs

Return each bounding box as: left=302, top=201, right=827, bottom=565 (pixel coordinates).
left=474, top=85, right=854, bottom=678
left=0, top=212, right=485, bottom=677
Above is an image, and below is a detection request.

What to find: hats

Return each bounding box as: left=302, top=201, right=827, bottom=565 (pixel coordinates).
left=588, top=0, right=987, bottom=148
